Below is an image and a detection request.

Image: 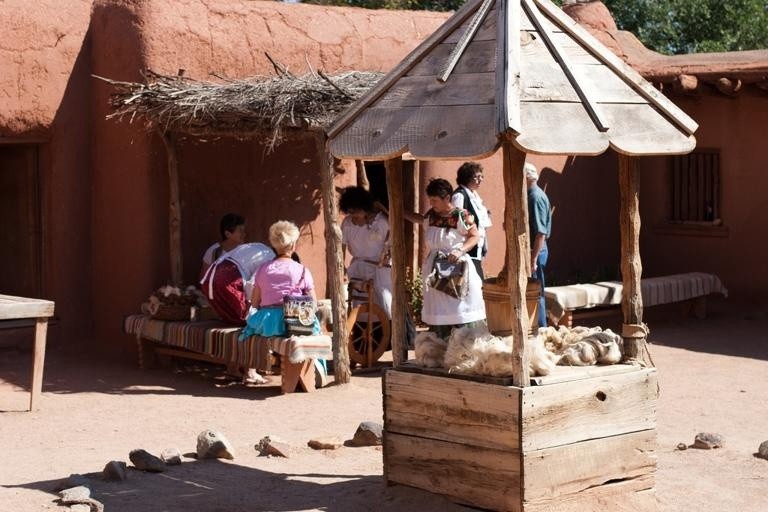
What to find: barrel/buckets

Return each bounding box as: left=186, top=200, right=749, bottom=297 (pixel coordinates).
left=482, top=278, right=542, bottom=336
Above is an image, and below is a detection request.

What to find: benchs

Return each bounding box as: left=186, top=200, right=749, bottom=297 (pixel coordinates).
left=125, top=315, right=332, bottom=392
left=542, top=272, right=727, bottom=329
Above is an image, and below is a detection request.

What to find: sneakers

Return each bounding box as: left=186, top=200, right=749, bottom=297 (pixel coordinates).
left=314, top=358, right=325, bottom=388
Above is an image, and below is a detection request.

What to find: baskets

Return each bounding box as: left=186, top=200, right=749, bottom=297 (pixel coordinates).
left=148, top=290, right=195, bottom=321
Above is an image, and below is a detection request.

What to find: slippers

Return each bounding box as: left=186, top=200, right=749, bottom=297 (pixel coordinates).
left=222, top=370, right=269, bottom=385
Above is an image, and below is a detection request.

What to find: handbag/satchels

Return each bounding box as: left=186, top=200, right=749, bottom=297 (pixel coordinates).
left=283, top=295, right=315, bottom=337
left=430, top=251, right=467, bottom=298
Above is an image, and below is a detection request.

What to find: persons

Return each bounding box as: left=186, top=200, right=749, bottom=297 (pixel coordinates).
left=239, top=220, right=330, bottom=390
left=403, top=178, right=489, bottom=343
left=451, top=161, right=493, bottom=282
left=339, top=186, right=394, bottom=369
left=522, top=162, right=552, bottom=328
left=197, top=240, right=300, bottom=385
left=199, top=211, right=246, bottom=279
